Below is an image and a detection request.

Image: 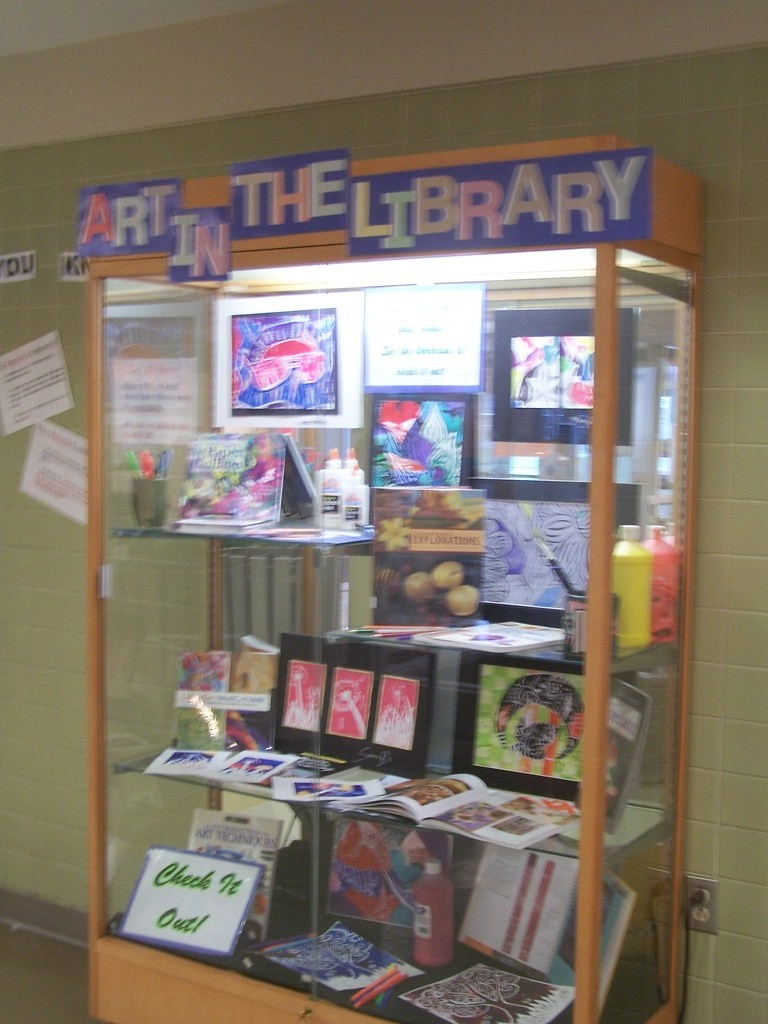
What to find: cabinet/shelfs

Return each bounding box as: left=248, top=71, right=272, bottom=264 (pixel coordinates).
left=86, top=135, right=704, bottom=1023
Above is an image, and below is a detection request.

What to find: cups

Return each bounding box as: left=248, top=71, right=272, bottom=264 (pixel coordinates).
left=129, top=476, right=170, bottom=528
left=565, top=592, right=620, bottom=660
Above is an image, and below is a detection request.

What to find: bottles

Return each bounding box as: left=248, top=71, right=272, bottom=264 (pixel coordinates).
left=640, top=525, right=680, bottom=644
left=411, top=857, right=454, bottom=968
left=612, top=525, right=651, bottom=651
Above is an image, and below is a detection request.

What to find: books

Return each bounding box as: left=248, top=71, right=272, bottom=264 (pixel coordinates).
left=412, top=621, right=566, bottom=653
left=342, top=774, right=577, bottom=851
left=604, top=677, right=652, bottom=835
left=187, top=808, right=285, bottom=942
left=172, top=514, right=274, bottom=535
left=169, top=650, right=279, bottom=751
left=455, top=839, right=638, bottom=1009
left=369, top=486, right=488, bottom=628
